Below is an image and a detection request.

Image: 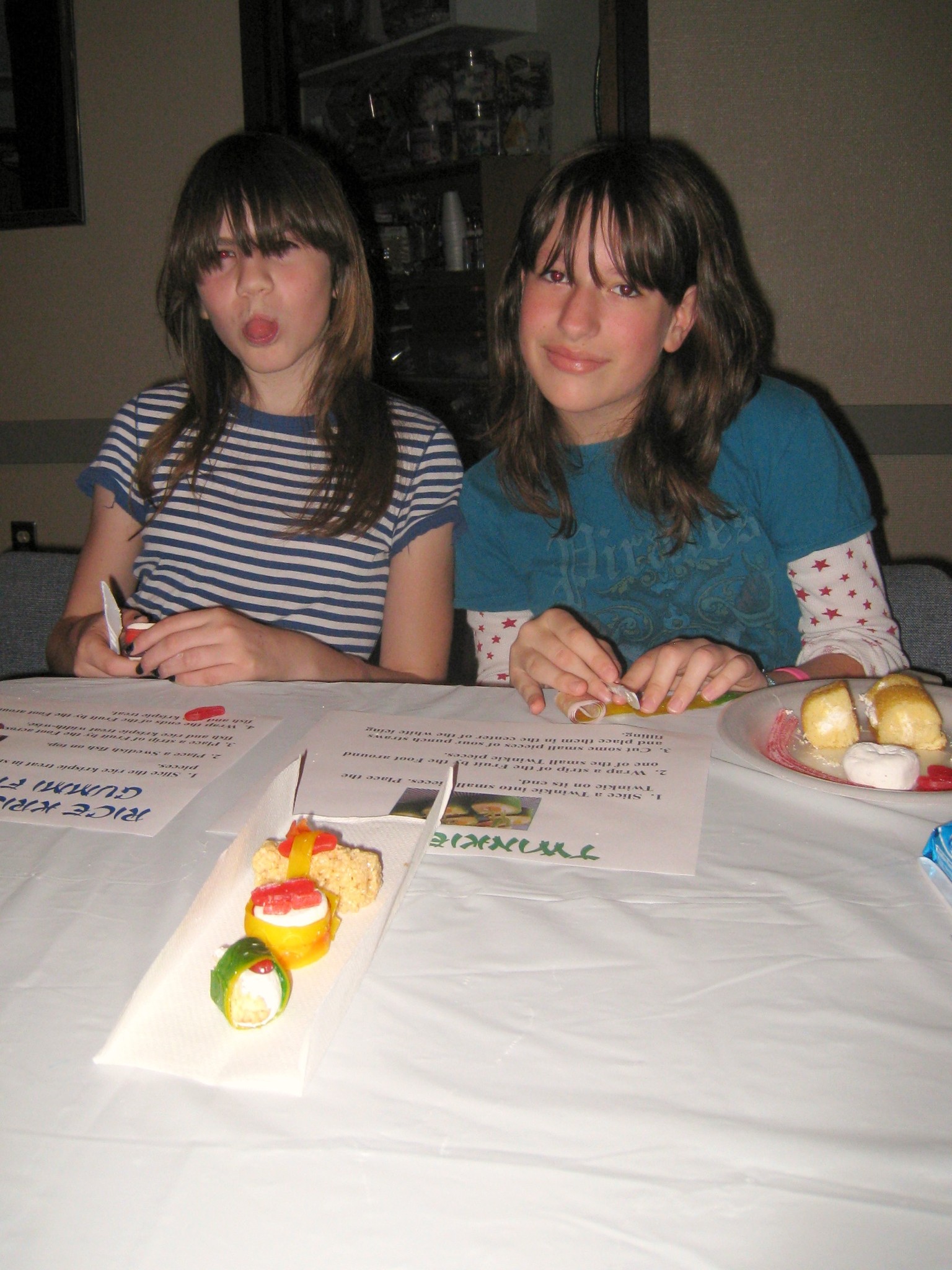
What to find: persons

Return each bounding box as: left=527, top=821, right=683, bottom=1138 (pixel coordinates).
left=39, top=125, right=466, bottom=689
left=451, top=139, right=905, bottom=717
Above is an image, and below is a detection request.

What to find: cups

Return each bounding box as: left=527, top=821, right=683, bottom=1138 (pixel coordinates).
left=439, top=189, right=469, bottom=270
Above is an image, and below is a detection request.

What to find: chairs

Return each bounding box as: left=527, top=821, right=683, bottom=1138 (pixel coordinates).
left=880, top=563, right=951, bottom=686
left=0, top=553, right=80, bottom=678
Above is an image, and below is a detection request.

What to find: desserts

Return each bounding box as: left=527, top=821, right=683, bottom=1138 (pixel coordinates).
left=243, top=877, right=342, bottom=969
left=253, top=819, right=384, bottom=911
left=210, top=938, right=291, bottom=1031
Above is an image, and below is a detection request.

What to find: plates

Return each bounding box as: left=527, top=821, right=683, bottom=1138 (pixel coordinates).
left=716, top=678, right=952, bottom=805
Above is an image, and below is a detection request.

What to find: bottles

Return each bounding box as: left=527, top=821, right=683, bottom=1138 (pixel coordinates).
left=471, top=214, right=485, bottom=270
left=365, top=44, right=538, bottom=159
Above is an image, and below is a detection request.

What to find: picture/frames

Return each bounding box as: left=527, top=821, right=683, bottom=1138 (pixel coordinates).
left=0, top=0, right=88, bottom=231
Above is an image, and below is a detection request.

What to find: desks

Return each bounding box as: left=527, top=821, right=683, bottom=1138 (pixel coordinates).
left=0, top=678, right=952, bottom=1270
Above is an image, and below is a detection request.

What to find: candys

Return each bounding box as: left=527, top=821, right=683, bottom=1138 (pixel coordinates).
left=917, top=765, right=952, bottom=793
left=184, top=706, right=226, bottom=721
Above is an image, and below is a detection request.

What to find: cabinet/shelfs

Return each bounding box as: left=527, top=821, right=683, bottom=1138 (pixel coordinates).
left=298, top=24, right=537, bottom=444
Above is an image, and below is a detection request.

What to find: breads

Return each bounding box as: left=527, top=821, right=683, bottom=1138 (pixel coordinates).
left=859, top=674, right=947, bottom=750
left=801, top=680, right=860, bottom=750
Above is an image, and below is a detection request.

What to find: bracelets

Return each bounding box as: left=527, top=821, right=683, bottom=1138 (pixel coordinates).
left=777, top=664, right=810, bottom=683
left=761, top=666, right=778, bottom=690
